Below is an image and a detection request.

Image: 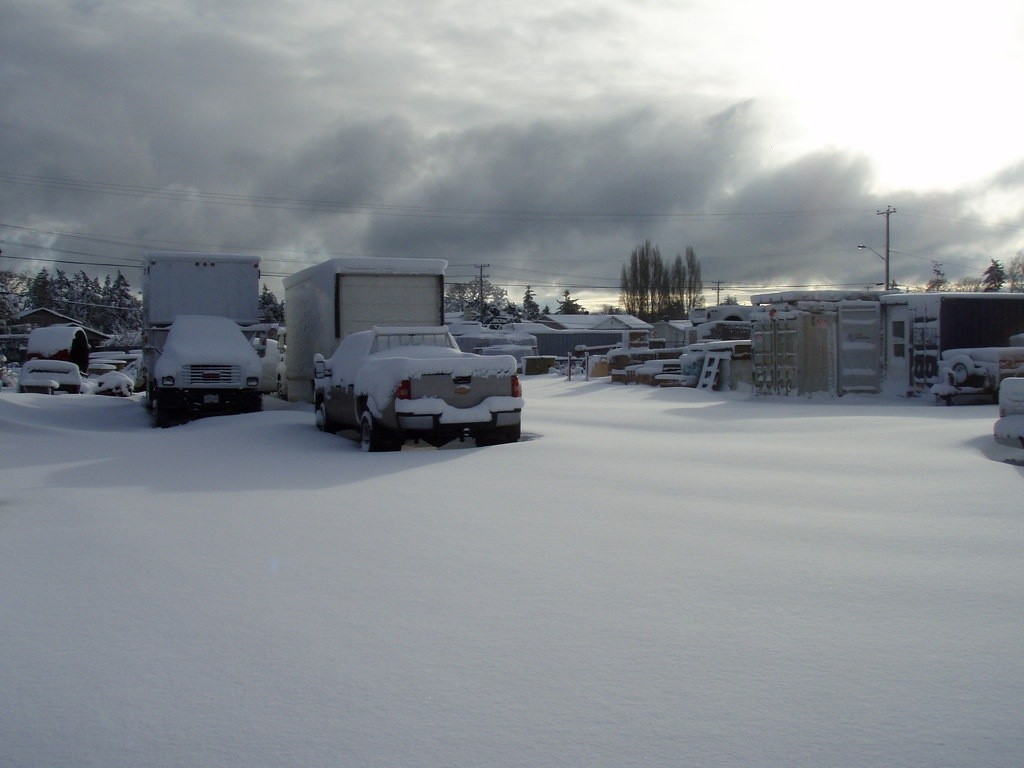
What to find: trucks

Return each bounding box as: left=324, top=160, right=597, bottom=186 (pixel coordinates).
left=278, top=253, right=448, bottom=402
left=140, top=249, right=265, bottom=429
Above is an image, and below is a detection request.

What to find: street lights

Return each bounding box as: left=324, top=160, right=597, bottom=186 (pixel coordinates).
left=858, top=244, right=890, bottom=290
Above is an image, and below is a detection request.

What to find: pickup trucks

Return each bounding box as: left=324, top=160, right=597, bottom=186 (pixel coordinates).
left=941, top=333, right=1024, bottom=405
left=314, top=327, right=522, bottom=452
